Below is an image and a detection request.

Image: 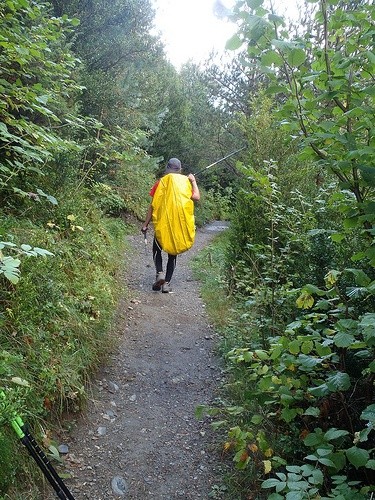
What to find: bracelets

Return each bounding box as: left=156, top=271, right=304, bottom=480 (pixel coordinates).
left=191, top=180, right=194, bottom=183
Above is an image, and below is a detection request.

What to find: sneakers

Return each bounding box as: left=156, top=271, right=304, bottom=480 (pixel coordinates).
left=162, top=282, right=172, bottom=293
left=153, top=272, right=165, bottom=291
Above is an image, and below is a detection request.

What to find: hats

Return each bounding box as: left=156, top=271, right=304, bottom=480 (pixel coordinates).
left=166, top=158, right=182, bottom=171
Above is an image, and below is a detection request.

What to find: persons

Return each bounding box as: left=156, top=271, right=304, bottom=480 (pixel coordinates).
left=140, top=158, right=200, bottom=293
